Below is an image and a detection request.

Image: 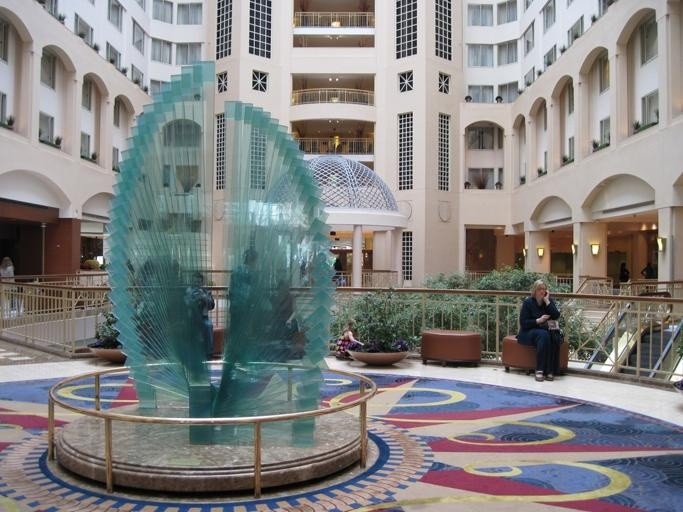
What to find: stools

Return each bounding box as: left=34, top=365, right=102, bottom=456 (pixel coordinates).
left=502, top=335, right=567, bottom=376
left=420, top=330, right=482, bottom=367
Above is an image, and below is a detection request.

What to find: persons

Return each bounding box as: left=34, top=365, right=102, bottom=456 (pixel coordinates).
left=619, top=262, right=629, bottom=282
left=0, top=256, right=16, bottom=283
left=336, top=277, right=346, bottom=286
left=517, top=279, right=561, bottom=382
left=183, top=273, right=215, bottom=360
left=267, top=280, right=298, bottom=340
left=229, top=250, right=259, bottom=328
left=641, top=262, right=653, bottom=292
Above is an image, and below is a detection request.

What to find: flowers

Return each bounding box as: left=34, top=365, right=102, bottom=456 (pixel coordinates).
left=88, top=294, right=120, bottom=348
left=345, top=288, right=416, bottom=352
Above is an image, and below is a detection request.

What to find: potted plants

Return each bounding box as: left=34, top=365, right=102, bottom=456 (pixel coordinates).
left=495, top=182, right=502, bottom=190
left=465, top=95, right=472, bottom=102
left=495, top=97, right=503, bottom=103
left=465, top=182, right=471, bottom=189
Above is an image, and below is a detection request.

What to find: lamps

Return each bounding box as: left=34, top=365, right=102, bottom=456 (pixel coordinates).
left=590, top=243, right=600, bottom=255
left=571, top=244, right=577, bottom=256
left=537, top=248, right=544, bottom=257
left=523, top=248, right=527, bottom=257
left=656, top=236, right=666, bottom=251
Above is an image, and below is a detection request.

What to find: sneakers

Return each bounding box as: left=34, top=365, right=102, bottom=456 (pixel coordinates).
left=534, top=369, right=553, bottom=381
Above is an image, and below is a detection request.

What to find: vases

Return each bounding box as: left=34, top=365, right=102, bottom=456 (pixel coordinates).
left=90, top=347, right=127, bottom=364
left=346, top=350, right=407, bottom=365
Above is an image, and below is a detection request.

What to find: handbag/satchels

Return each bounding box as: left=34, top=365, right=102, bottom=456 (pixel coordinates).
left=538, top=319, right=560, bottom=331
